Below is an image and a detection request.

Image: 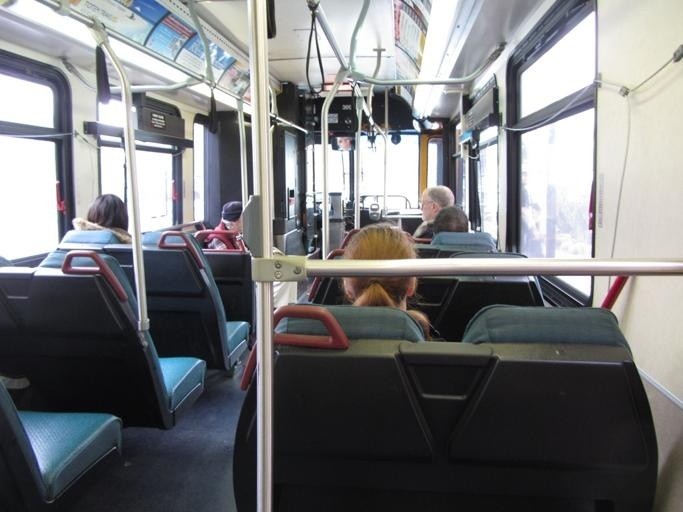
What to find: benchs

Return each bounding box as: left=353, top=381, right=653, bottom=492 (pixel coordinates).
left=343, top=229, right=498, bottom=256
left=229, top=304, right=657, bottom=512
left=1, top=249, right=207, bottom=432
left=312, top=254, right=548, bottom=344
left=1, top=377, right=122, bottom=512
left=61, top=229, right=249, bottom=371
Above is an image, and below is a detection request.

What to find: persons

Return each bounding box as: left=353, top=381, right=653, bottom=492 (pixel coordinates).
left=434, top=206, right=471, bottom=240
left=411, top=184, right=455, bottom=238
left=285, top=188, right=294, bottom=217
left=335, top=136, right=354, bottom=150
left=206, top=201, right=298, bottom=311
left=339, top=225, right=446, bottom=344
left=71, top=194, right=141, bottom=247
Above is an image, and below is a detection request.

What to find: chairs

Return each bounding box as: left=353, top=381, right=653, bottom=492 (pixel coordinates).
left=382, top=209, right=423, bottom=235
left=196, top=228, right=243, bottom=252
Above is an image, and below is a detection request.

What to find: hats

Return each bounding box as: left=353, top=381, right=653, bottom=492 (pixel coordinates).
left=221, top=201, right=242, bottom=220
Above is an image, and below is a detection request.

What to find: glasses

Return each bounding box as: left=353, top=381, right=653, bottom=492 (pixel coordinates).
left=418, top=200, right=433, bottom=209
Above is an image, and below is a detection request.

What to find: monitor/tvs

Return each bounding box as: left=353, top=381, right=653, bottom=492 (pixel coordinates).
left=331, top=136, right=355, bottom=150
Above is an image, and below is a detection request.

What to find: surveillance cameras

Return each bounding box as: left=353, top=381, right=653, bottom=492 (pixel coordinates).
left=390, top=134, right=401, bottom=144
